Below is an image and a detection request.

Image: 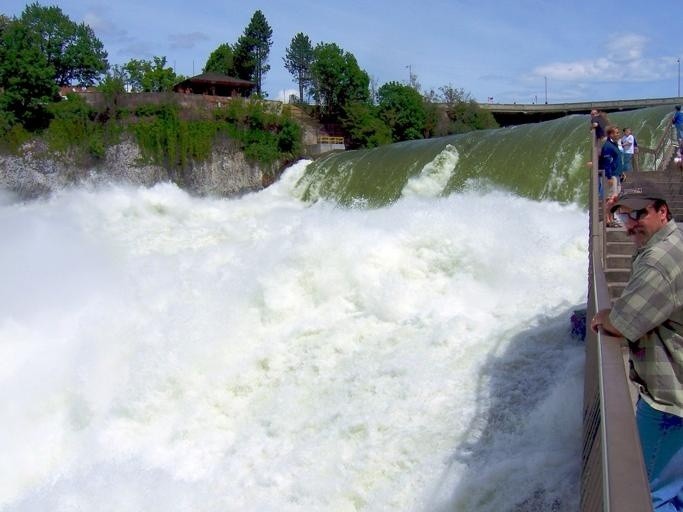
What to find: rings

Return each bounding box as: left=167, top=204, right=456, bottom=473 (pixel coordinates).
left=593, top=318, right=594, bottom=320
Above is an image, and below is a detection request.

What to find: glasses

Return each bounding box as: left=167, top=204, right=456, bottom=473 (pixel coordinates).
left=616, top=209, right=649, bottom=223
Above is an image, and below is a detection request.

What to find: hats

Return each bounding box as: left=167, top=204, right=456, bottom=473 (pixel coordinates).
left=611, top=179, right=667, bottom=210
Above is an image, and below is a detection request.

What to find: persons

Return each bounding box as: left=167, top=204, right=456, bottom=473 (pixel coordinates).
left=601, top=126, right=622, bottom=225
left=591, top=180, right=683, bottom=512
left=591, top=109, right=611, bottom=144
left=672, top=104, right=683, bottom=168
left=621, top=128, right=638, bottom=171
